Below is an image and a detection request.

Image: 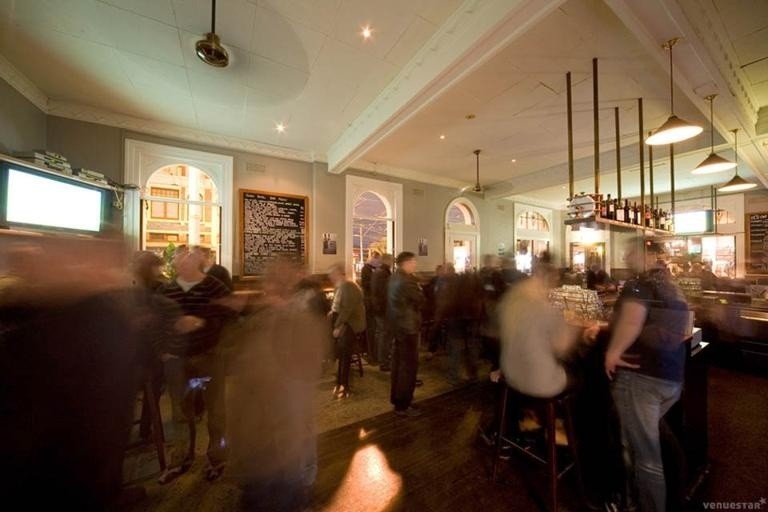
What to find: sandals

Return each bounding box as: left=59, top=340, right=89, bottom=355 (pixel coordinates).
left=332, top=383, right=359, bottom=399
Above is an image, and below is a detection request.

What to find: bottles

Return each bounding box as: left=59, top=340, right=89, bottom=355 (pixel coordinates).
left=599, top=193, right=671, bottom=234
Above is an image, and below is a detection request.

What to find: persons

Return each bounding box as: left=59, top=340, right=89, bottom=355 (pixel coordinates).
left=0, top=222, right=767, bottom=512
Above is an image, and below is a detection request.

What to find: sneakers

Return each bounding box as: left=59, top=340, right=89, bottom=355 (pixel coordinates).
left=393, top=405, right=424, bottom=418
left=206, top=462, right=226, bottom=485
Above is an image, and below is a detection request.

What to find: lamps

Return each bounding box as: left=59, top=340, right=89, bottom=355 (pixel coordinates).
left=473, top=150, right=483, bottom=194
left=717, top=130, right=758, bottom=192
left=645, top=36, right=704, bottom=146
left=692, top=94, right=738, bottom=175
left=195, top=0, right=230, bottom=67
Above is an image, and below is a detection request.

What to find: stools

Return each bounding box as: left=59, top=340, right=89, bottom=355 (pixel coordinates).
left=357, top=328, right=374, bottom=365
left=490, top=384, right=580, bottom=512
left=125, top=369, right=167, bottom=477
